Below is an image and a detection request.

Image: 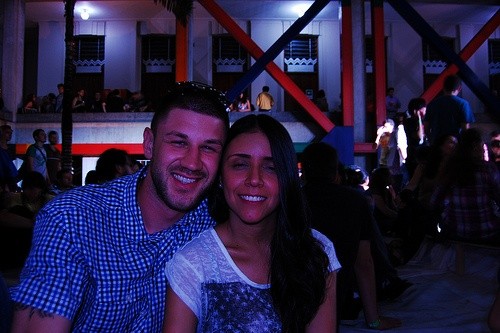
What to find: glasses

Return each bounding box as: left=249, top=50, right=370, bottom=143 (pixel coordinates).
left=174, top=79, right=222, bottom=100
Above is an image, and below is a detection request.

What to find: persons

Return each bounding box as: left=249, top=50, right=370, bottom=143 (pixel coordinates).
left=0, top=73, right=500, bottom=333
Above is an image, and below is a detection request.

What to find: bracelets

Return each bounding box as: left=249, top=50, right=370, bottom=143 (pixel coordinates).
left=367, top=319, right=381, bottom=329
